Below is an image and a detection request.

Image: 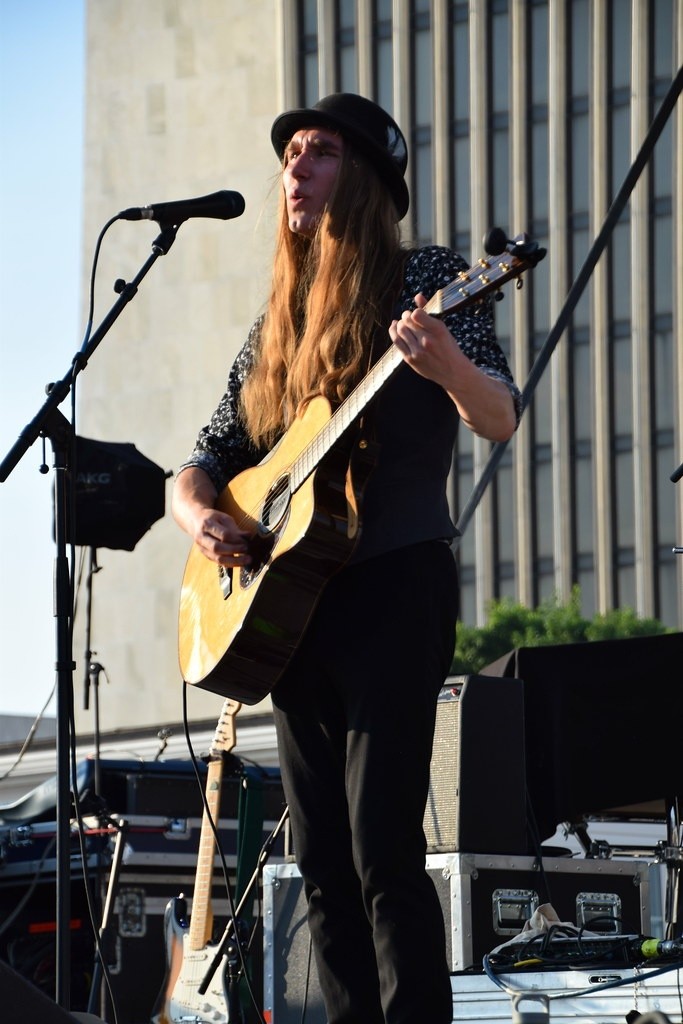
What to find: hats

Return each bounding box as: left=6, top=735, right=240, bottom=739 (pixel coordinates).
left=271, top=93, right=410, bottom=223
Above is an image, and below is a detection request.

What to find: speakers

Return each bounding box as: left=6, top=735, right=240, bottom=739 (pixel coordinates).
left=420, top=675, right=529, bottom=856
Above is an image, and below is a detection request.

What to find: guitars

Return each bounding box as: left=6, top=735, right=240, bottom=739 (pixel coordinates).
left=176, top=228, right=548, bottom=703
left=149, top=696, right=241, bottom=1024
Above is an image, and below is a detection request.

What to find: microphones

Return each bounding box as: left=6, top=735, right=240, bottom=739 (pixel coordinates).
left=121, top=190, right=245, bottom=221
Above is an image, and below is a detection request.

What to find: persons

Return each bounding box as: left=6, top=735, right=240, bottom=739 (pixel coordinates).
left=170, top=90, right=524, bottom=1024
